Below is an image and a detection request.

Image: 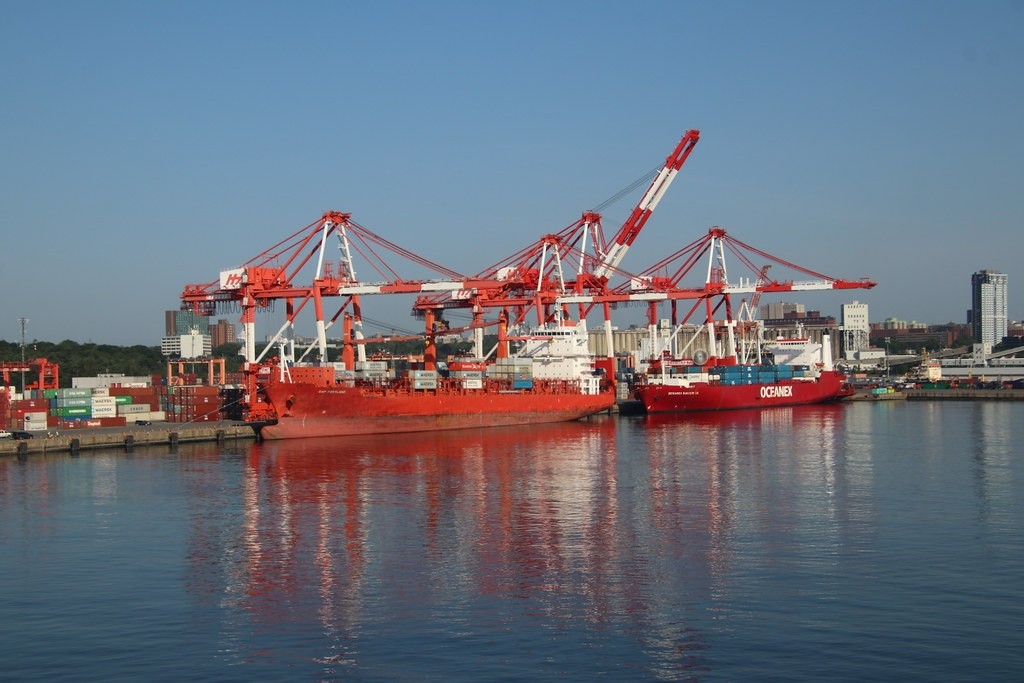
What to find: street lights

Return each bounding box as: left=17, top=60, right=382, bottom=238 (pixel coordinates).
left=885, top=336, right=891, bottom=378
left=190, top=328, right=198, bottom=373
left=16, top=318, right=29, bottom=400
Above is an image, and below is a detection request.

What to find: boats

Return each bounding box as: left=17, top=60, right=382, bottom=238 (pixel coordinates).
left=617, top=351, right=857, bottom=417
left=239, top=333, right=618, bottom=440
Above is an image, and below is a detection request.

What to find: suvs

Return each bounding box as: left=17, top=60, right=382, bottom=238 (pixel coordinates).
left=12, top=430, right=33, bottom=442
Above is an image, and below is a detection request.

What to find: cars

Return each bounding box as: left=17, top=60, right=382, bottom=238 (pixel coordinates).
left=135, top=419, right=151, bottom=426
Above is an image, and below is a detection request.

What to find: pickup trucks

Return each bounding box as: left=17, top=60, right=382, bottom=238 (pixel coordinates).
left=0, top=430, right=11, bottom=437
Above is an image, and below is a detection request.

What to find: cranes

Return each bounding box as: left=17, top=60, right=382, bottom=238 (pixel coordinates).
left=745, top=265, right=771, bottom=321
